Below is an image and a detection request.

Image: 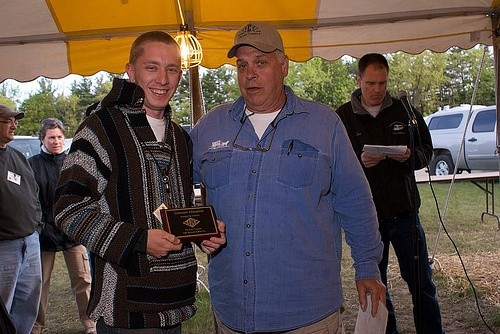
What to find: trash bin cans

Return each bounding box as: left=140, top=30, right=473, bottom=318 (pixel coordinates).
left=183, top=126, right=191, bottom=132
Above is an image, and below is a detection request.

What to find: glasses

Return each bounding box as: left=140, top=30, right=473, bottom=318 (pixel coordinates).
left=233, top=112, right=278, bottom=152
left=0, top=121, right=18, bottom=126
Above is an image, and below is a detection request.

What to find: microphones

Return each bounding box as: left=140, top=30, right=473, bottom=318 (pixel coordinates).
left=398, top=90, right=417, bottom=124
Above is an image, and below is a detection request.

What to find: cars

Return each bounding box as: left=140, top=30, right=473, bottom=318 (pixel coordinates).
left=7, top=136, right=42, bottom=161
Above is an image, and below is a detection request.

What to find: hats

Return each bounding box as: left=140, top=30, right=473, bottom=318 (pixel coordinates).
left=227, top=23, right=285, bottom=58
left=0, top=105, right=24, bottom=119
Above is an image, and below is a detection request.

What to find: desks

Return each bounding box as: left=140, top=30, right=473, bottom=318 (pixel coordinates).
left=416, top=171, right=500, bottom=229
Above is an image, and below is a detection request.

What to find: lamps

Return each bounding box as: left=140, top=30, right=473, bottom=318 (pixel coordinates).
left=173, top=0, right=203, bottom=69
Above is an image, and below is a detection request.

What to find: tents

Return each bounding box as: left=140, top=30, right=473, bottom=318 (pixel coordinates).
left=0, top=0, right=500, bottom=207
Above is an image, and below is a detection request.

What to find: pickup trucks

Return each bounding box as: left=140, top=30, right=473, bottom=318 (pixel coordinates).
left=426, top=104, right=497, bottom=183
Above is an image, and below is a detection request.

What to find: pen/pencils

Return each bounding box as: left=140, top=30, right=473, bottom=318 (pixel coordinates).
left=287, top=140, right=294, bottom=155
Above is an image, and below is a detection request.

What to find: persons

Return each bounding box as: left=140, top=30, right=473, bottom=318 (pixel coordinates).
left=188, top=23, right=387, bottom=334
left=27, top=118, right=96, bottom=334
left=0, top=106, right=45, bottom=334
left=54, top=31, right=227, bottom=334
left=335, top=53, right=445, bottom=334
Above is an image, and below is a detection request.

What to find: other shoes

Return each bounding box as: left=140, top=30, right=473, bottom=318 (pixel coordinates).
left=82, top=319, right=97, bottom=334
left=31, top=322, right=43, bottom=334
